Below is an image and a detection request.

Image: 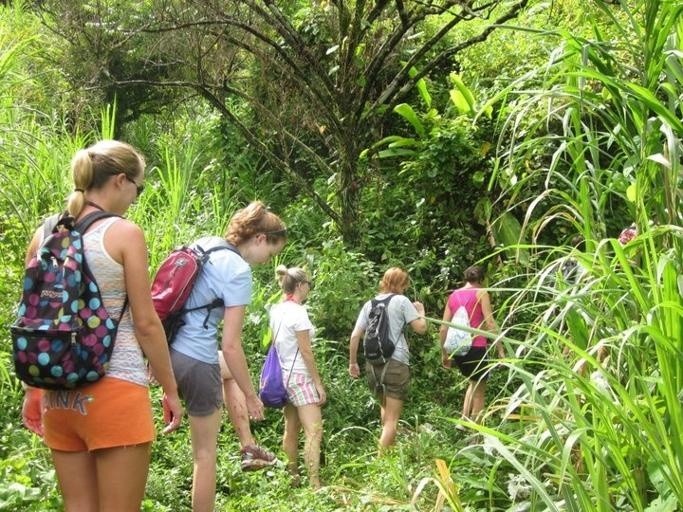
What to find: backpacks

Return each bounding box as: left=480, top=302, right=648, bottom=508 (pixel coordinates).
left=363, top=293, right=406, bottom=366
left=151, top=246, right=243, bottom=345
left=10, top=212, right=137, bottom=390
left=443, top=289, right=479, bottom=357
left=258, top=317, right=299, bottom=408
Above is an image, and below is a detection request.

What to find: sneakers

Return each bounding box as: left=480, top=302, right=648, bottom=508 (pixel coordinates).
left=240, top=446, right=278, bottom=473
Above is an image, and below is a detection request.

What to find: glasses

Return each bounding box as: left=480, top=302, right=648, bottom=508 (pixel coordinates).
left=127, top=178, right=145, bottom=195
left=256, top=227, right=288, bottom=238
left=305, top=281, right=311, bottom=285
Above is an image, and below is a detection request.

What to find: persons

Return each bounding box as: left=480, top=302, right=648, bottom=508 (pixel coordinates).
left=264, top=262, right=329, bottom=496
left=15, top=135, right=184, bottom=512
left=152, top=196, right=293, bottom=512
left=344, top=264, right=428, bottom=461
left=436, top=266, right=507, bottom=435
